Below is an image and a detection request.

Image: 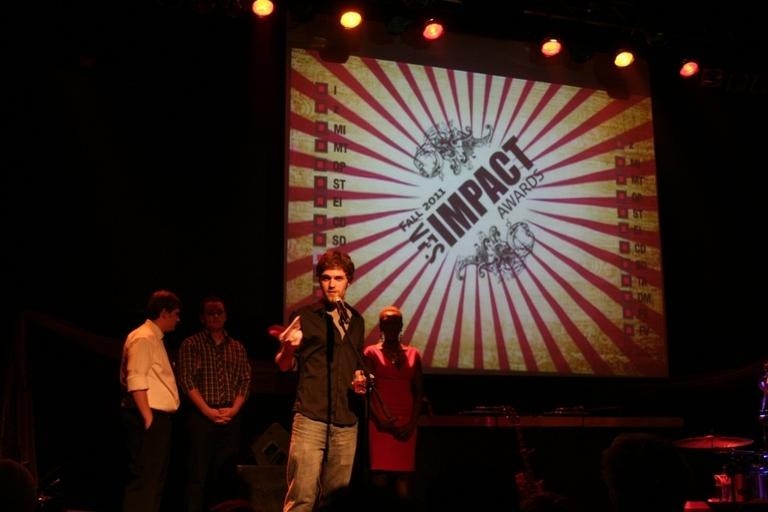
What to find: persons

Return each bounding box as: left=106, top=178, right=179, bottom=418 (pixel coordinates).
left=363, top=305, right=423, bottom=472
left=119, top=289, right=181, bottom=511
left=175, top=294, right=253, bottom=512
left=1, top=434, right=681, bottom=511
left=273, top=248, right=376, bottom=512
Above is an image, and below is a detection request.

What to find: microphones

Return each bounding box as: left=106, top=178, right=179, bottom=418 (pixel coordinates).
left=333, top=295, right=350, bottom=323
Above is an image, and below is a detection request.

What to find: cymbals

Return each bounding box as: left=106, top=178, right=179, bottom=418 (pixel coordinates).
left=672, top=436, right=756, bottom=451
